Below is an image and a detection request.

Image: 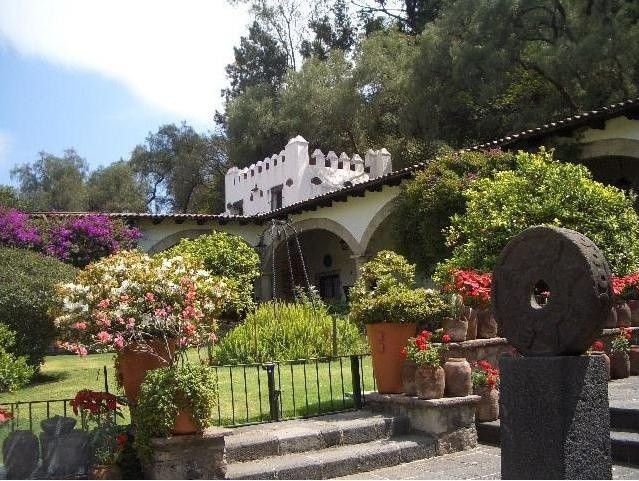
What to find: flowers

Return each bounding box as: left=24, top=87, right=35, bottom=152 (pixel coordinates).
left=588, top=339, right=606, bottom=354
left=611, top=271, right=639, bottom=302
left=49, top=252, right=218, bottom=358
left=471, top=361, right=500, bottom=388
left=449, top=268, right=493, bottom=310
left=398, top=330, right=450, bottom=370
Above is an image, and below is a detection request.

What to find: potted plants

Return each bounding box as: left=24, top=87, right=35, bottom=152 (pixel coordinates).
left=444, top=296, right=468, bottom=342
left=608, top=335, right=630, bottom=378
left=133, top=365, right=218, bottom=467
left=348, top=249, right=442, bottom=394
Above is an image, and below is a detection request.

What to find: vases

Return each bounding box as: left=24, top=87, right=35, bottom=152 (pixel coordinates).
left=606, top=299, right=638, bottom=329
left=469, top=310, right=497, bottom=339
left=630, top=345, right=638, bottom=375
left=118, top=338, right=176, bottom=404
left=442, top=356, right=470, bottom=398
left=416, top=365, right=444, bottom=399
left=595, top=352, right=611, bottom=380
left=476, top=387, right=500, bottom=421
